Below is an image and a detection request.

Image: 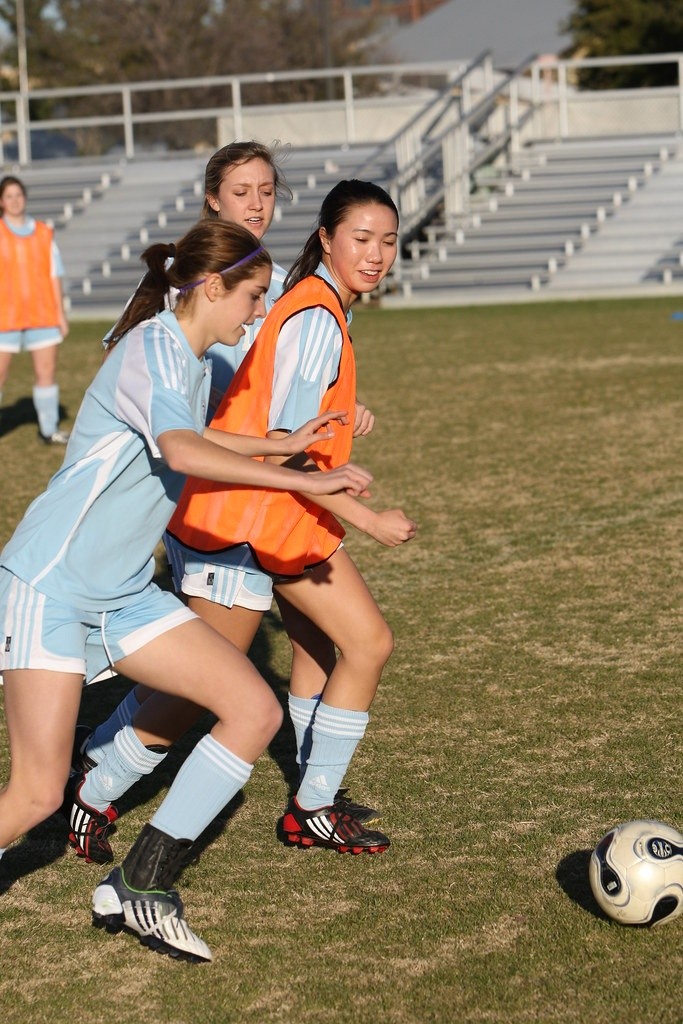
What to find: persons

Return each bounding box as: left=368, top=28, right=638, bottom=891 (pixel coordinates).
left=1, top=220, right=372, bottom=963
left=68, top=141, right=378, bottom=821
left=0, top=175, right=69, bottom=445
left=58, top=181, right=400, bottom=863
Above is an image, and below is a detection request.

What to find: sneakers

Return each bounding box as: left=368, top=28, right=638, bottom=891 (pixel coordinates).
left=57, top=772, right=118, bottom=865
left=70, top=725, right=99, bottom=774
left=282, top=794, right=392, bottom=855
left=92, top=866, right=212, bottom=964
left=287, top=784, right=381, bottom=825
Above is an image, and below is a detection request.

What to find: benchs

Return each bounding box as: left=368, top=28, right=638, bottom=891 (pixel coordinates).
left=0, top=133, right=683, bottom=316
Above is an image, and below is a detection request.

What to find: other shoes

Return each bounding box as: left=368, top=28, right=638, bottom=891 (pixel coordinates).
left=36, top=431, right=68, bottom=445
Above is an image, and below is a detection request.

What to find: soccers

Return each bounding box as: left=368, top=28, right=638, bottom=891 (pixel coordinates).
left=587, top=820, right=683, bottom=928
